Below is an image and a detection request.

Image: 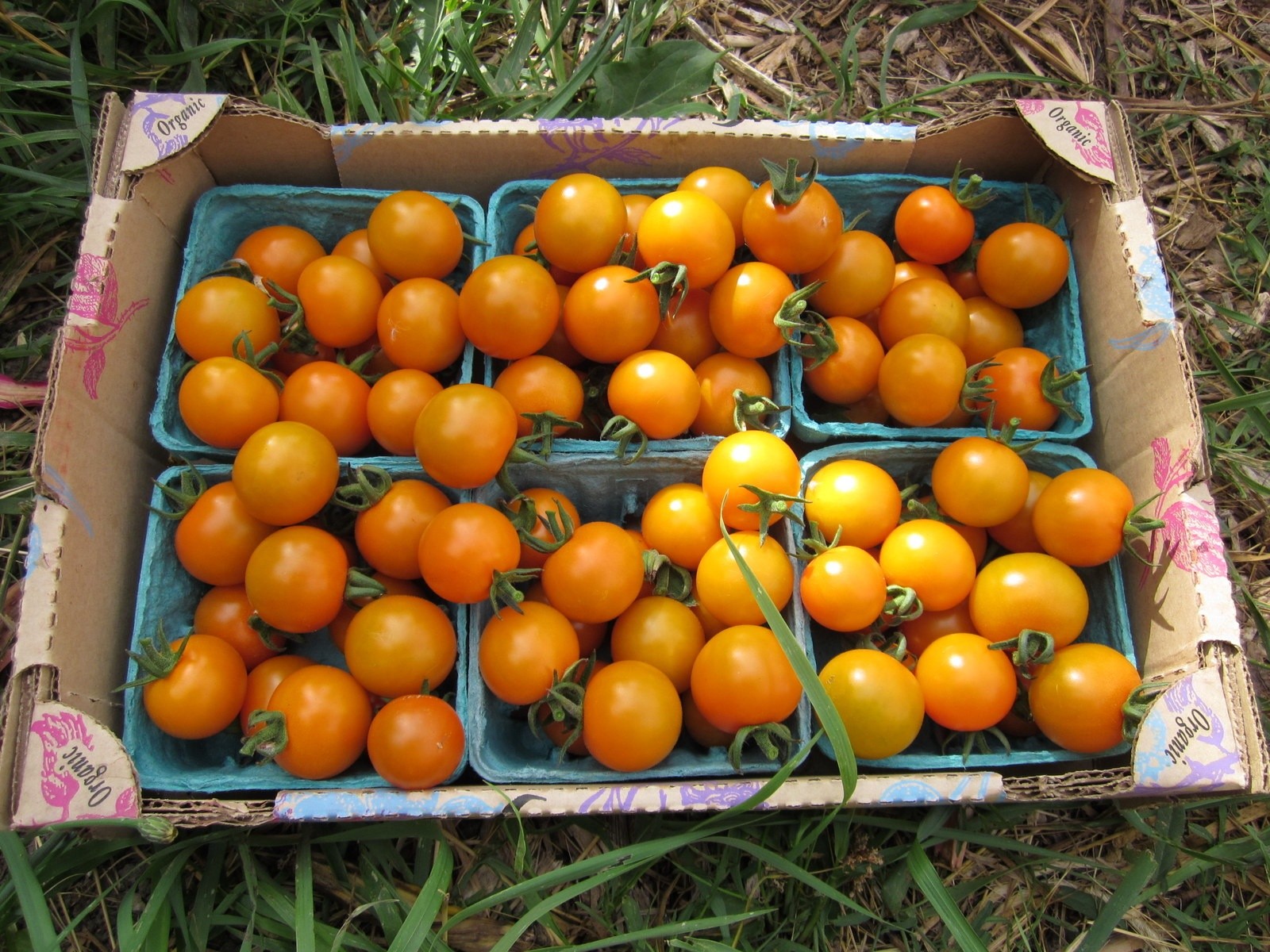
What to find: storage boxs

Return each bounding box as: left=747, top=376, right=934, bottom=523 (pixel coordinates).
left=788, top=442, right=1137, bottom=768
left=485, top=176, right=791, bottom=451
left=469, top=453, right=810, bottom=782
left=789, top=172, right=1094, bottom=442
left=149, top=184, right=486, bottom=465
left=122, top=465, right=469, bottom=792
left=0, top=91, right=1270, bottom=827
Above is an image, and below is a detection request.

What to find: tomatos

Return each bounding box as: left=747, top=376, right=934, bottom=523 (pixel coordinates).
left=105, top=159, right=1171, bottom=776
